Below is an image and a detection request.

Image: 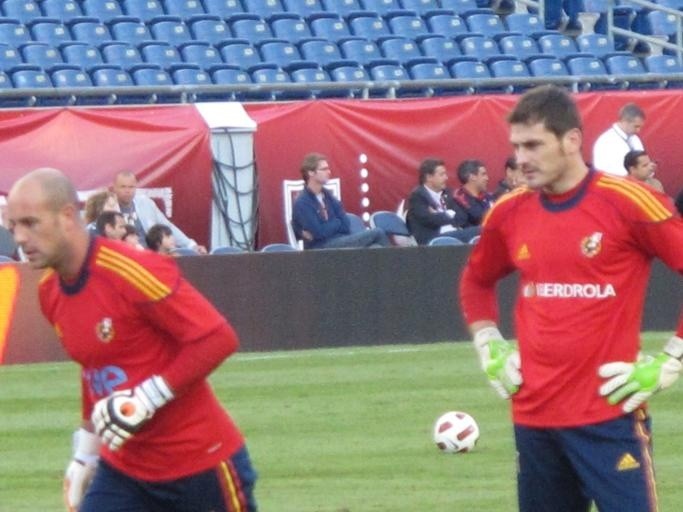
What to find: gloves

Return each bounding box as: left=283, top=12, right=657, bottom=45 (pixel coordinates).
left=472, top=326, right=524, bottom=400
left=597, top=334, right=683, bottom=413
left=91, top=374, right=176, bottom=452
left=61, top=426, right=104, bottom=512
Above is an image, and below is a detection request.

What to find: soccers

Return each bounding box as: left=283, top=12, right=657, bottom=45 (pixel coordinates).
left=434, top=412, right=479, bottom=454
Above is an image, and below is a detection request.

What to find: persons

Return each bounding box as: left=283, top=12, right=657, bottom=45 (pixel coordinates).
left=0, top=170, right=207, bottom=262
left=8, top=168, right=259, bottom=511
left=406, top=101, right=683, bottom=242
left=460, top=83, right=683, bottom=512
left=293, top=152, right=391, bottom=249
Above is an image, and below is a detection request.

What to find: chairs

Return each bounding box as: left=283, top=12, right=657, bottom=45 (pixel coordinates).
left=468, top=235, right=481, bottom=243
left=0, top=1, right=682, bottom=113
left=343, top=213, right=368, bottom=232
left=210, top=245, right=243, bottom=254
left=169, top=248, right=201, bottom=258
left=262, top=243, right=298, bottom=252
left=289, top=219, right=307, bottom=252
left=1, top=226, right=23, bottom=265
left=370, top=211, right=419, bottom=249
left=429, top=236, right=465, bottom=245
